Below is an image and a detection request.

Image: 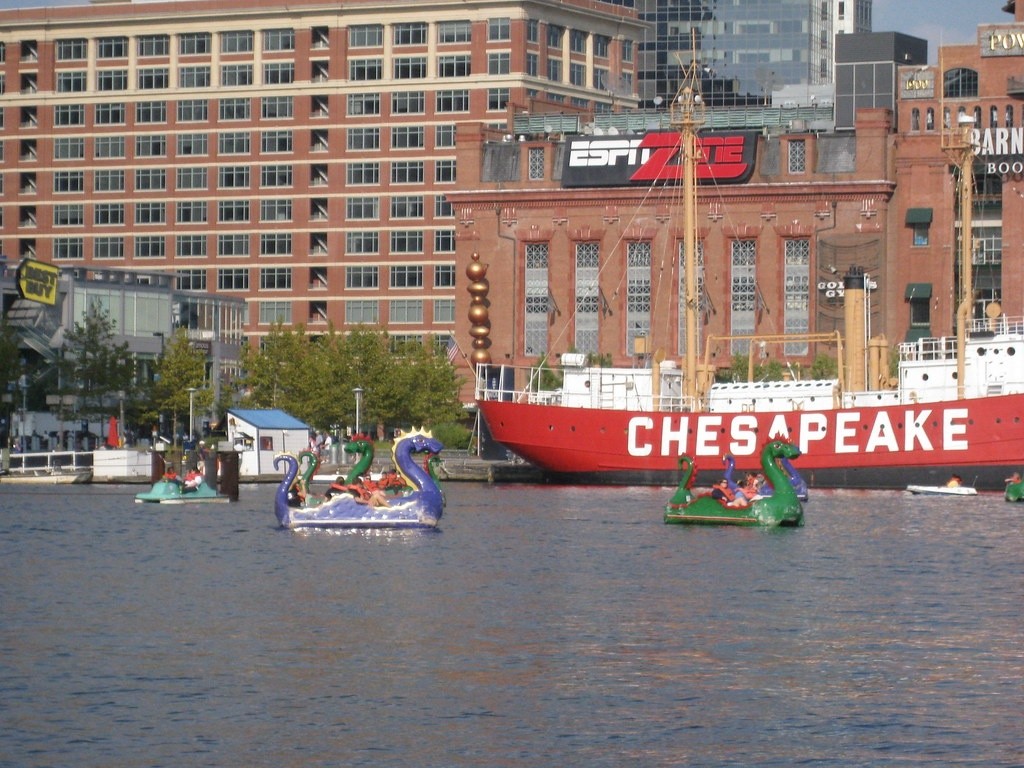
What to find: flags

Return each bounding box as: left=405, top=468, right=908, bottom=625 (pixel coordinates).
left=446, top=337, right=459, bottom=362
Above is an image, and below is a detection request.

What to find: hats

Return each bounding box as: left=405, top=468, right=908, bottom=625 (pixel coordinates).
left=387, top=469, right=396, bottom=474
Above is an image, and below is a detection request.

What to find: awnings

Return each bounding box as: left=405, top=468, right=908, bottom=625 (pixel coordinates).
left=905, top=283, right=932, bottom=298
left=904, top=329, right=931, bottom=345
left=905, top=208, right=933, bottom=224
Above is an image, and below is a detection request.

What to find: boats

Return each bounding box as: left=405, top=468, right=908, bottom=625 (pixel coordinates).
left=719, top=445, right=809, bottom=503
left=662, top=433, right=806, bottom=528
left=134, top=478, right=232, bottom=505
left=0, top=469, right=93, bottom=486
left=905, top=483, right=978, bottom=495
left=1003, top=474, right=1024, bottom=503
left=272, top=425, right=446, bottom=533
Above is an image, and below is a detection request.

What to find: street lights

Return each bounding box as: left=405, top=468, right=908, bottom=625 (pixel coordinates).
left=151, top=330, right=165, bottom=438
left=186, top=387, right=198, bottom=440
left=18, top=375, right=30, bottom=453
left=116, top=388, right=127, bottom=449
left=350, top=388, right=366, bottom=436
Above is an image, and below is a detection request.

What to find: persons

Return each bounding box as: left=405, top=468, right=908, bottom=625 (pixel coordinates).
left=99, top=432, right=209, bottom=494
left=1005, top=473, right=1021, bottom=485
left=13, top=439, right=23, bottom=453
left=946, top=474, right=962, bottom=488
left=287, top=477, right=306, bottom=509
left=712, top=471, right=764, bottom=509
left=309, top=429, right=373, bottom=465
left=323, top=469, right=406, bottom=509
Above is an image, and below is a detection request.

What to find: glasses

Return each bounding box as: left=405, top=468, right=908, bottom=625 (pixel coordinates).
left=365, top=478, right=371, bottom=481
left=722, top=482, right=727, bottom=484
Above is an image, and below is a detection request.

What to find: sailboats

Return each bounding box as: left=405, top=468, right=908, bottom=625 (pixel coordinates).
left=473, top=23, right=1024, bottom=492
